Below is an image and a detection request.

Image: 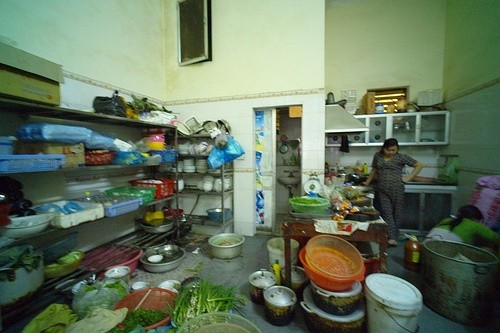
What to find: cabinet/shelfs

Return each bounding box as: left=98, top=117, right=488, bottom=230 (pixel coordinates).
left=399, top=184, right=457, bottom=235
left=153, top=134, right=235, bottom=233
left=0, top=96, right=180, bottom=323
left=326, top=111, right=450, bottom=146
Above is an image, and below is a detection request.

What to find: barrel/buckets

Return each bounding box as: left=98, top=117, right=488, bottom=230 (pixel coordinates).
left=265, top=237, right=300, bottom=269
left=421, top=234, right=500, bottom=327
left=404, top=235, right=421, bottom=274
left=363, top=272, right=423, bottom=333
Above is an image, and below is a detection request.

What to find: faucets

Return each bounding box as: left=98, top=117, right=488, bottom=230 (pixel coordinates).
left=288, top=172, right=295, bottom=177
left=402, top=166, right=411, bottom=176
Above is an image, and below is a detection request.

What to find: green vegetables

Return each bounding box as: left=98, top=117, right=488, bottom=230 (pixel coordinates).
left=74, top=282, right=126, bottom=319
left=105, top=307, right=168, bottom=333
left=0, top=248, right=41, bottom=281
left=292, top=196, right=321, bottom=209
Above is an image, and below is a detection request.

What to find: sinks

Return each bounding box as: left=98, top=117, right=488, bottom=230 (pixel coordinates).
left=277, top=177, right=300, bottom=185
left=403, top=177, right=433, bottom=183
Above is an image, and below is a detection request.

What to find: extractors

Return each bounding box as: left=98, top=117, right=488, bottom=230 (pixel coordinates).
left=325, top=104, right=369, bottom=136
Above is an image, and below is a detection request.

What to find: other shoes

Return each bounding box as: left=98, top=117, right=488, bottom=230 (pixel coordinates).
left=386, top=241, right=397, bottom=248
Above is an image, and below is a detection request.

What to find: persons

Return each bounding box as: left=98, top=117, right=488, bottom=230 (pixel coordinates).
left=421, top=205, right=500, bottom=271
left=362, top=138, right=424, bottom=245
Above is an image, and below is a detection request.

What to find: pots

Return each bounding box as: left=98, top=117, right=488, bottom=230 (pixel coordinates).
left=248, top=265, right=366, bottom=333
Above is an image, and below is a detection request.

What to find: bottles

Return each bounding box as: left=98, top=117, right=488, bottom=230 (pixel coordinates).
left=325, top=161, right=329, bottom=174
left=363, top=163, right=367, bottom=174
left=288, top=152, right=296, bottom=165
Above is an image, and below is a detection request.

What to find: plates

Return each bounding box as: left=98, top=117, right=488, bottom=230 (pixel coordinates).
left=132, top=281, right=146, bottom=289
left=158, top=279, right=181, bottom=293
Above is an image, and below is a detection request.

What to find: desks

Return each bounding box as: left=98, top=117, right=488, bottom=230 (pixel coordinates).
left=281, top=215, right=389, bottom=289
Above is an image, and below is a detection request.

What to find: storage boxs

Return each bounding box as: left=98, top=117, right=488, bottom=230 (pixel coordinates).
left=0, top=142, right=179, bottom=228
left=418, top=89, right=444, bottom=106
left=0, top=42, right=64, bottom=106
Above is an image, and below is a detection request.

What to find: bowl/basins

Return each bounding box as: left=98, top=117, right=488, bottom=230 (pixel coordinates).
left=207, top=207, right=232, bottom=222
left=300, top=246, right=365, bottom=290
left=0, top=213, right=55, bottom=239
left=176, top=144, right=230, bottom=193
left=208, top=232, right=245, bottom=259
left=375, top=104, right=384, bottom=114
left=78, top=245, right=143, bottom=279
left=289, top=197, right=329, bottom=214
left=397, top=95, right=407, bottom=113
left=140, top=244, right=186, bottom=273
left=139, top=221, right=175, bottom=233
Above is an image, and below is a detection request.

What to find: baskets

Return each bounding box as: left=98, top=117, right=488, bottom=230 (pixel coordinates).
left=115, top=288, right=178, bottom=331
left=305, top=234, right=364, bottom=278
left=105, top=185, right=157, bottom=204
left=0, top=153, right=64, bottom=173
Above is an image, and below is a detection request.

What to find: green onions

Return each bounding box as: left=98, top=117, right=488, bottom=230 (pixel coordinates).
left=172, top=275, right=247, bottom=328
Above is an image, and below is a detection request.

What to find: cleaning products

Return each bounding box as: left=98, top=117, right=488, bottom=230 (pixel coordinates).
left=363, top=163, right=368, bottom=174
